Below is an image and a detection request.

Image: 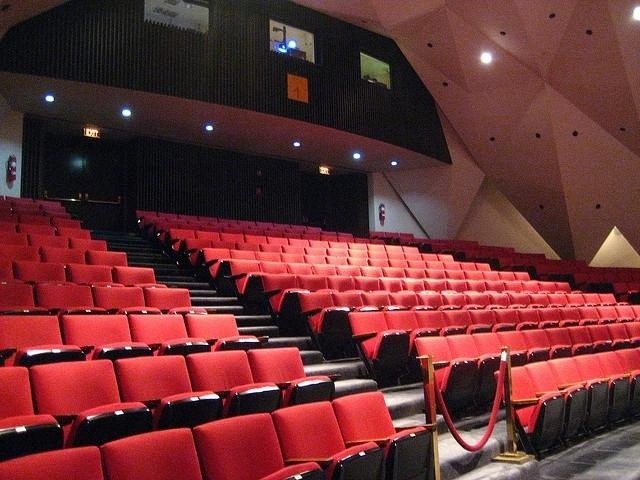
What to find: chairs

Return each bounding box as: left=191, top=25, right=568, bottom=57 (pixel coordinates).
left=0, top=193, right=640, bottom=480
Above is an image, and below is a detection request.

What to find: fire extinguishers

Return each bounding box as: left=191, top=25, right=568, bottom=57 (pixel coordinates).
left=379, top=204, right=385, bottom=220
left=8, top=154, right=16, bottom=181
